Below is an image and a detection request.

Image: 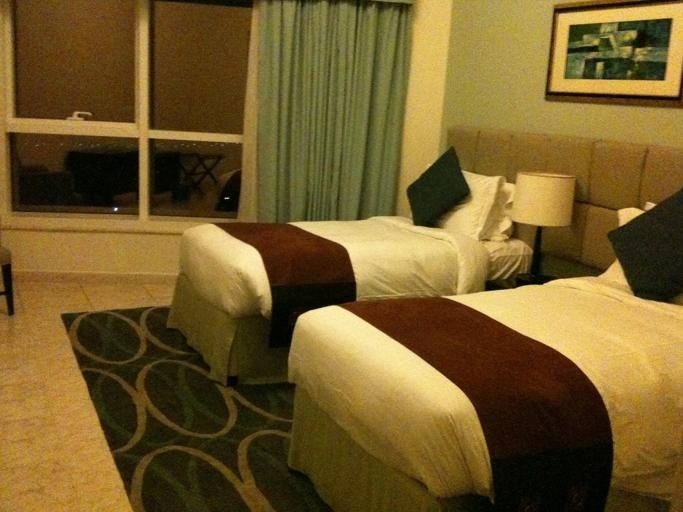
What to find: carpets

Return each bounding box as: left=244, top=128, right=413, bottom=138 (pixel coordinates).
left=59, top=303, right=333, bottom=512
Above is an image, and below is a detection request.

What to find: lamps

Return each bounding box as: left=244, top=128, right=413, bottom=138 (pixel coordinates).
left=510, top=170, right=577, bottom=286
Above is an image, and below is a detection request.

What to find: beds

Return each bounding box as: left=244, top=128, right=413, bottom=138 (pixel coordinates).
left=169, top=219, right=538, bottom=386
left=285, top=272, right=682, bottom=511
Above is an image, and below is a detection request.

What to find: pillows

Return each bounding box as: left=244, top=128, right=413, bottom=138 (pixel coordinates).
left=601, top=185, right=683, bottom=304
left=404, top=145, right=517, bottom=244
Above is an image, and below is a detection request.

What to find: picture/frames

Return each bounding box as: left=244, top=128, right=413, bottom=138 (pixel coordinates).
left=545, top=1, right=683, bottom=99
